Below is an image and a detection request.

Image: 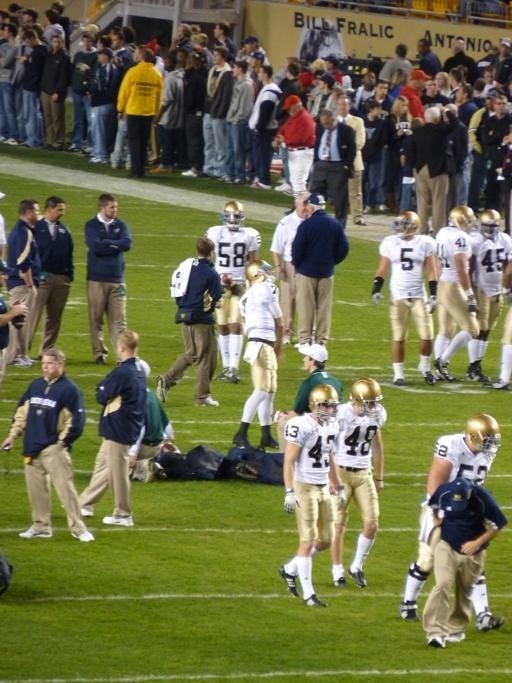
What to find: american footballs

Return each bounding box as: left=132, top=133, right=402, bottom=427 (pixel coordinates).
left=12, top=301, right=28, bottom=329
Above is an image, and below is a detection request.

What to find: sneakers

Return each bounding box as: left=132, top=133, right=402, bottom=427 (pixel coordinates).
left=274, top=182, right=293, bottom=197
left=156, top=375, right=168, bottom=401
left=0, top=136, right=18, bottom=145
left=445, top=633, right=466, bottom=642
left=418, top=363, right=434, bottom=385
left=214, top=367, right=240, bottom=383
left=393, top=375, right=405, bottom=385
left=234, top=432, right=251, bottom=448
left=433, top=357, right=456, bottom=382
left=428, top=636, right=446, bottom=648
left=466, top=360, right=491, bottom=383
left=249, top=177, right=272, bottom=189
left=401, top=600, right=418, bottom=621
left=149, top=166, right=173, bottom=173
left=483, top=378, right=510, bottom=390
left=278, top=564, right=299, bottom=597
left=476, top=607, right=504, bottom=631
left=304, top=594, right=328, bottom=608
left=194, top=394, right=219, bottom=407
left=61, top=503, right=94, bottom=517
left=89, top=156, right=108, bottom=164
left=334, top=576, right=347, bottom=587
left=71, top=530, right=95, bottom=543
left=19, top=528, right=52, bottom=537
left=219, top=175, right=244, bottom=184
left=9, top=358, right=37, bottom=368
left=180, top=169, right=198, bottom=177
left=261, top=438, right=279, bottom=448
left=102, top=516, right=134, bottom=527
left=348, top=564, right=367, bottom=588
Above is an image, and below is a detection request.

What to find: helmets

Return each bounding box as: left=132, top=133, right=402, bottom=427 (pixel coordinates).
left=478, top=209, right=501, bottom=239
left=245, top=259, right=276, bottom=285
left=308, top=384, right=341, bottom=423
left=351, top=378, right=385, bottom=418
left=222, top=202, right=246, bottom=228
left=448, top=206, right=478, bottom=236
left=465, top=413, right=502, bottom=455
left=393, top=210, right=421, bottom=238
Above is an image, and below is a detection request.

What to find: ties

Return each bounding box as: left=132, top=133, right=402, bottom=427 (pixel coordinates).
left=325, top=128, right=334, bottom=161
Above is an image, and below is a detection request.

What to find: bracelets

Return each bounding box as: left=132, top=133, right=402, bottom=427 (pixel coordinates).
left=374, top=477, right=383, bottom=482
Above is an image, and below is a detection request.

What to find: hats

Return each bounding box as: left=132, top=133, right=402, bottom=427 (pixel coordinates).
left=410, top=69, right=431, bottom=81
left=247, top=53, right=264, bottom=63
left=450, top=478, right=469, bottom=512
left=281, top=95, right=301, bottom=109
left=322, top=55, right=339, bottom=67
left=317, top=73, right=334, bottom=88
left=502, top=36, right=511, bottom=47
left=298, top=344, right=328, bottom=363
left=484, top=87, right=502, bottom=100
left=241, top=37, right=258, bottom=44
left=21, top=10, right=38, bottom=18
left=304, top=194, right=326, bottom=204
left=96, top=48, right=112, bottom=56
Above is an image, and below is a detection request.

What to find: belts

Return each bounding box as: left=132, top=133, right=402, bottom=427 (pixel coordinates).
left=142, top=440, right=161, bottom=447
left=249, top=338, right=274, bottom=347
left=312, top=484, right=326, bottom=486
left=339, top=465, right=366, bottom=473
left=288, top=146, right=315, bottom=151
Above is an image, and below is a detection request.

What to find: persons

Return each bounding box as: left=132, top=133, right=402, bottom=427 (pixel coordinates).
left=231, top=258, right=285, bottom=450
left=277, top=383, right=339, bottom=610
left=395, top=412, right=506, bottom=634
left=155, top=234, right=223, bottom=410
left=2, top=346, right=98, bottom=544
left=128, top=382, right=175, bottom=483
left=1, top=192, right=74, bottom=368
left=76, top=330, right=149, bottom=527
left=1, top=0, right=512, bottom=238
left=324, top=376, right=390, bottom=594
left=372, top=207, right=512, bottom=391
left=207, top=200, right=271, bottom=384
left=85, top=193, right=132, bottom=365
left=270, top=191, right=349, bottom=348
left=423, top=476, right=509, bottom=650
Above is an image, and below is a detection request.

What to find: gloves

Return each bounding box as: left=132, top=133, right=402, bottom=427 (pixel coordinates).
left=424, top=296, right=436, bottom=313
left=283, top=488, right=300, bottom=512
left=465, top=288, right=480, bottom=317
left=337, top=485, right=347, bottom=510
left=372, top=292, right=383, bottom=304
